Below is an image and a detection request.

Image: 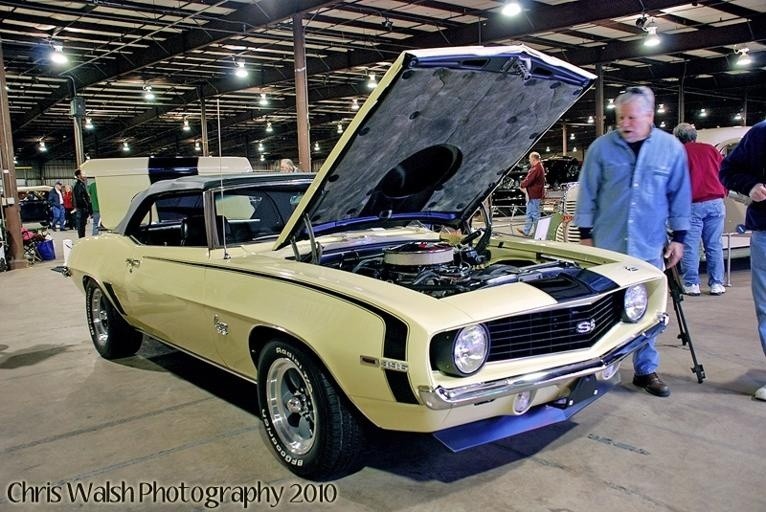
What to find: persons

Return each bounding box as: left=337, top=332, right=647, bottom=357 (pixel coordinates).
left=48, top=169, right=100, bottom=237
left=573, top=85, right=693, bottom=398
left=280, top=159, right=304, bottom=172
left=717, top=119, right=766, bottom=402
left=517, top=151, right=545, bottom=239
left=673, top=122, right=729, bottom=296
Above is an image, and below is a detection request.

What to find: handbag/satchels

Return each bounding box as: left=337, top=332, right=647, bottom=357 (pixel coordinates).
left=36, top=238, right=55, bottom=260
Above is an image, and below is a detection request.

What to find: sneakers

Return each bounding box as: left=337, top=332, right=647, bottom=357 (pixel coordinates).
left=755, top=385, right=765, bottom=400
left=710, top=283, right=727, bottom=295
left=682, top=282, right=700, bottom=296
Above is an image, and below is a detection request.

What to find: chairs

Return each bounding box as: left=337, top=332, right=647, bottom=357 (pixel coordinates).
left=177, top=214, right=236, bottom=248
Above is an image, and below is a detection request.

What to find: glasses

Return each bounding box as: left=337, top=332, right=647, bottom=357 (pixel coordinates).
left=615, top=88, right=650, bottom=110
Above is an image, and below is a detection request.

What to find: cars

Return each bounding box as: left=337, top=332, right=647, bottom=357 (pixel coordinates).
left=0, top=185, right=72, bottom=227
left=473, top=137, right=752, bottom=265
left=51, top=44, right=670, bottom=482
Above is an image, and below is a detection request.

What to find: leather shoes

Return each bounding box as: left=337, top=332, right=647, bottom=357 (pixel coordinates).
left=632, top=372, right=670, bottom=398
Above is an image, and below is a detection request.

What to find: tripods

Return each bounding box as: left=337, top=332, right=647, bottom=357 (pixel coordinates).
left=662, top=218, right=708, bottom=384
